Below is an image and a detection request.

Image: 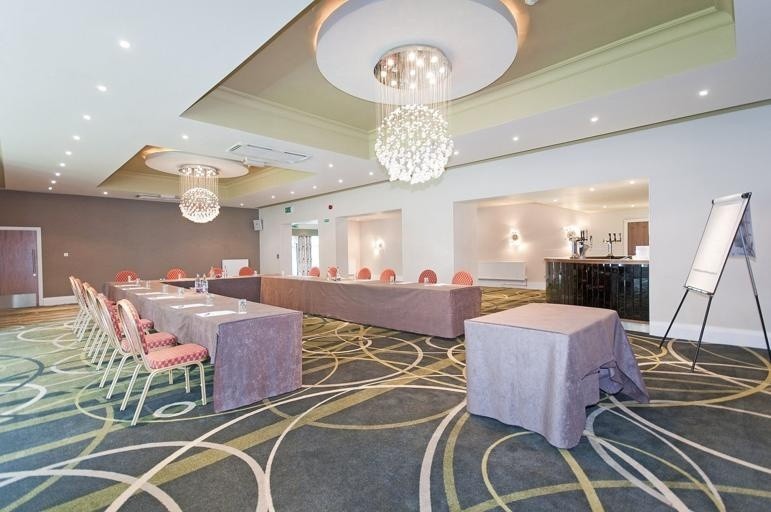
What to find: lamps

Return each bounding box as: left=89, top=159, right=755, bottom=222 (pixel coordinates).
left=374, top=46, right=455, bottom=185
left=179, top=166, right=221, bottom=223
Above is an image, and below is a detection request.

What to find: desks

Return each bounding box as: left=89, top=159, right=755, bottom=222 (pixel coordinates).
left=102, top=281, right=303, bottom=413
left=261, top=274, right=482, bottom=339
left=464, top=302, right=650, bottom=449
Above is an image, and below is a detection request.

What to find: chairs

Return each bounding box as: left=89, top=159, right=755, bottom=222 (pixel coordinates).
left=358, top=268, right=371, bottom=280
left=379, top=269, right=395, bottom=281
left=95, top=294, right=177, bottom=400
left=85, top=286, right=155, bottom=370
left=451, top=271, right=472, bottom=286
left=308, top=268, right=320, bottom=276
left=70, top=275, right=92, bottom=342
left=418, top=269, right=437, bottom=283
left=115, top=299, right=209, bottom=426
left=104, top=267, right=262, bottom=304
left=327, top=268, right=338, bottom=278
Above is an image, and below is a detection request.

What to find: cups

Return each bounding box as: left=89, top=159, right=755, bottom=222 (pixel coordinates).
left=128, top=276, right=246, bottom=312
left=389, top=275, right=394, bottom=284
left=424, top=277, right=429, bottom=287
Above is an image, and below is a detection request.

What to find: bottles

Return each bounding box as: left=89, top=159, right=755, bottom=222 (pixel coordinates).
left=210, top=264, right=215, bottom=278
left=335, top=266, right=340, bottom=282
left=327, top=267, right=331, bottom=280
left=224, top=265, right=227, bottom=277
left=195, top=272, right=208, bottom=293
